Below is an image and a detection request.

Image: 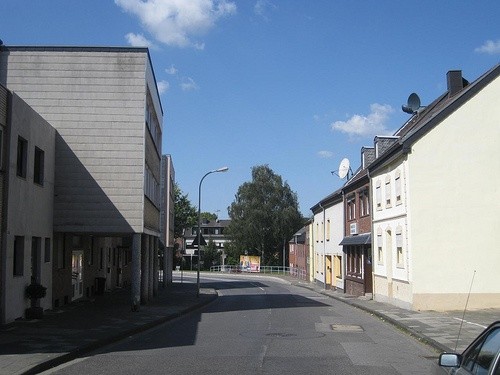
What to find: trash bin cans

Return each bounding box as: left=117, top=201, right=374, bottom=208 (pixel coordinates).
left=94, top=276, right=108, bottom=296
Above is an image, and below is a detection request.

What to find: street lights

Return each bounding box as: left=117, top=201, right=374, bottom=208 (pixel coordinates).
left=195, top=167, right=230, bottom=299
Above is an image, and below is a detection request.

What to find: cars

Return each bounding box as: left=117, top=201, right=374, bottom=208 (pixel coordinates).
left=438, top=321, right=500, bottom=375
